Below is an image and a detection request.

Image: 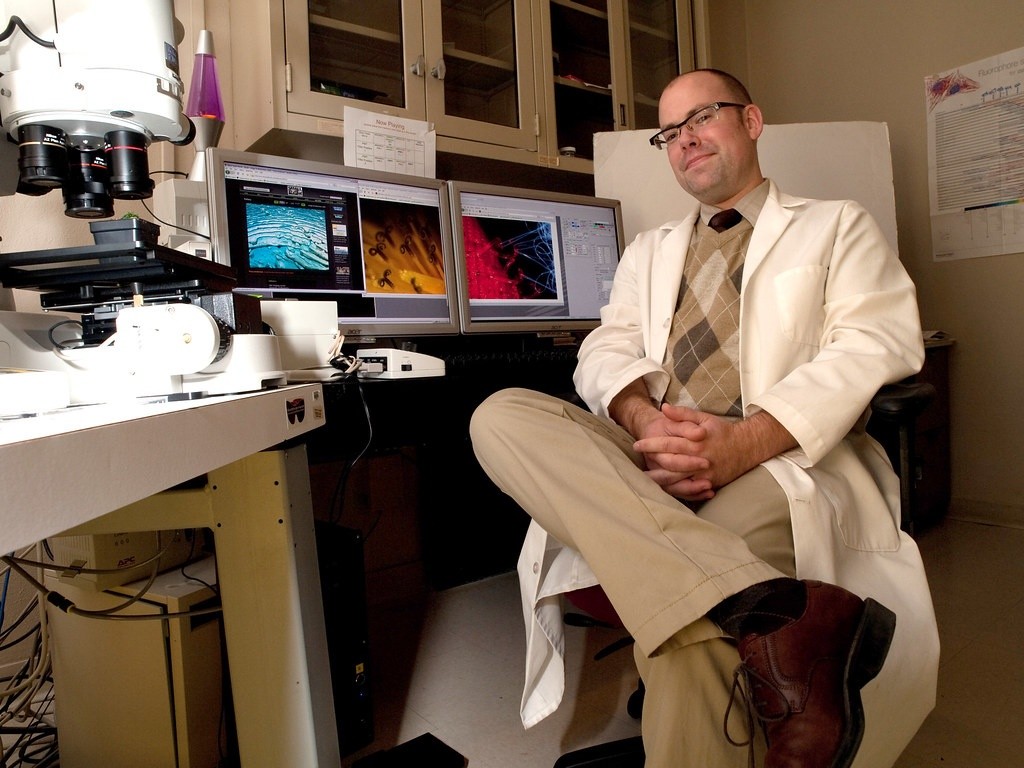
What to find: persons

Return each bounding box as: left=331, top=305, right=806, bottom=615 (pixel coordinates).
left=469, top=65, right=927, bottom=762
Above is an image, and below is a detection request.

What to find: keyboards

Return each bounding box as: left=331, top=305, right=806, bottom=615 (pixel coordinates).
left=441, top=351, right=577, bottom=367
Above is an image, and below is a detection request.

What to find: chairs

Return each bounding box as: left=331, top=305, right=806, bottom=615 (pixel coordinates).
left=553, top=373, right=939, bottom=768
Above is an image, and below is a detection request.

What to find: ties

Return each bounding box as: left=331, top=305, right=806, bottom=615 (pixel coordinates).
left=710, top=208, right=743, bottom=234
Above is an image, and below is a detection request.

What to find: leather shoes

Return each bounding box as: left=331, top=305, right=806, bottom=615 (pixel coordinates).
left=722, top=579, right=897, bottom=768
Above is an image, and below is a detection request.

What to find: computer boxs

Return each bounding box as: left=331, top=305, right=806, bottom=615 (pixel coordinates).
left=215, top=519, right=377, bottom=768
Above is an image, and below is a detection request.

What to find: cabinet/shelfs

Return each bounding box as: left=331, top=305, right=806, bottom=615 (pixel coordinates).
left=180, top=1, right=710, bottom=179
left=888, top=335, right=956, bottom=528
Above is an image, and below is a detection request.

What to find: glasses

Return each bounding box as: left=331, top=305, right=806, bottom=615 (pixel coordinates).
left=649, top=101, right=749, bottom=152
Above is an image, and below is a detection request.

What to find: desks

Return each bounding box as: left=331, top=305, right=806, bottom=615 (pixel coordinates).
left=0, top=379, right=343, bottom=768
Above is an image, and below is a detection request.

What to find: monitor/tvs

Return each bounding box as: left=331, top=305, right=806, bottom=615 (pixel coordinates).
left=446, top=180, right=626, bottom=338
left=205, top=147, right=459, bottom=360
